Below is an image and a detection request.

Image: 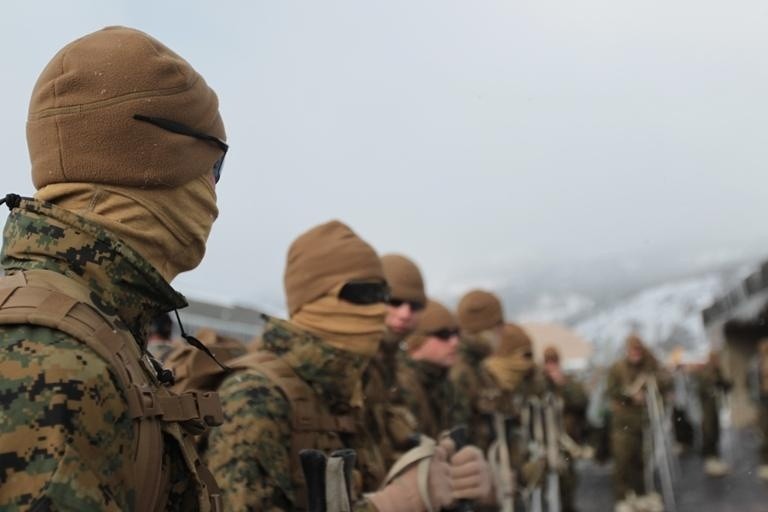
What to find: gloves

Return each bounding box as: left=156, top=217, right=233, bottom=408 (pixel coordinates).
left=363, top=436, right=496, bottom=512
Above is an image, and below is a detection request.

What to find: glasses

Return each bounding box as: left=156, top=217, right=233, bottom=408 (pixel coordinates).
left=132, top=113, right=229, bottom=184
left=422, top=327, right=461, bottom=342
left=327, top=281, right=390, bottom=306
left=389, top=300, right=424, bottom=311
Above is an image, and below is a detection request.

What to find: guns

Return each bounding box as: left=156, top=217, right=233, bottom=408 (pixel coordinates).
left=440, top=425, right=478, bottom=511
left=298, top=449, right=357, bottom=511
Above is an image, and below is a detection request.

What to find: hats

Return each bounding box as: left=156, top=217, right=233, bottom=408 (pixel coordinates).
left=453, top=291, right=503, bottom=332
left=379, top=254, right=427, bottom=306
left=407, top=300, right=460, bottom=350
left=285, top=221, right=386, bottom=318
left=488, top=322, right=531, bottom=358
left=26, top=26, right=227, bottom=188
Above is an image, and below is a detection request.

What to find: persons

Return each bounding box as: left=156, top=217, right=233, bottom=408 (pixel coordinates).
left=0, top=24, right=224, bottom=511
